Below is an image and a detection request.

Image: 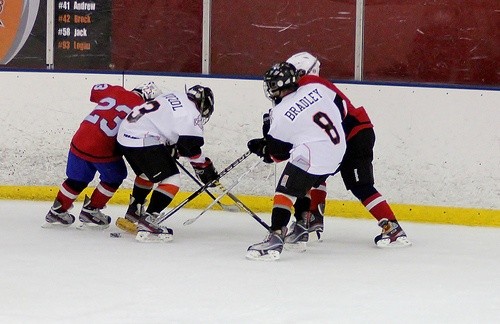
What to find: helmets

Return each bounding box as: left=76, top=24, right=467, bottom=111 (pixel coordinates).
left=263, top=62, right=298, bottom=106
left=134, top=81, right=162, bottom=101
left=285, top=52, right=321, bottom=77
left=187, top=84, right=215, bottom=125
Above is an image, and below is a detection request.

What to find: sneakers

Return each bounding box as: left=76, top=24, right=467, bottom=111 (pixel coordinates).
left=125, top=194, right=148, bottom=225
left=41, top=198, right=75, bottom=227
left=76, top=194, right=111, bottom=230
left=245, top=226, right=288, bottom=261
left=135, top=212, right=173, bottom=243
left=375, top=218, right=412, bottom=247
left=308, top=204, right=325, bottom=241
left=284, top=212, right=308, bottom=252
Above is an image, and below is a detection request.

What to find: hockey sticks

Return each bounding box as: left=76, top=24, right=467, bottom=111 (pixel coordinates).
left=215, top=181, right=307, bottom=252
left=175, top=159, right=242, bottom=213
left=115, top=150, right=253, bottom=234
left=183, top=156, right=264, bottom=226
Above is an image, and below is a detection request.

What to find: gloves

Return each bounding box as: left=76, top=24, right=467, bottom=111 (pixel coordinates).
left=247, top=139, right=273, bottom=163
left=262, top=113, right=270, bottom=139
left=190, top=156, right=219, bottom=188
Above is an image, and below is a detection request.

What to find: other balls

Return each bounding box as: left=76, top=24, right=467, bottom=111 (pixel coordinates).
left=110, top=233, right=120, bottom=237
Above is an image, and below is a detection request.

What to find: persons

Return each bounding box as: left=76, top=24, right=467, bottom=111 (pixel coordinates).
left=286, top=51, right=412, bottom=247
left=246, top=61, right=346, bottom=260
left=118, top=85, right=223, bottom=241
left=41, top=80, right=162, bottom=231
